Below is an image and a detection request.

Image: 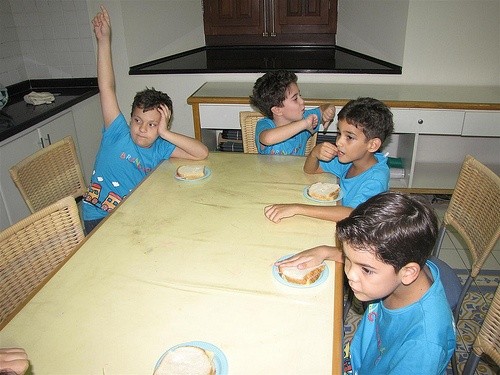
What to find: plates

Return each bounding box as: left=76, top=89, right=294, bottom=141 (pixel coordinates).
left=304, top=183, right=343, bottom=202
left=273, top=253, right=330, bottom=287
left=153, top=342, right=228, bottom=375
left=174, top=167, right=210, bottom=181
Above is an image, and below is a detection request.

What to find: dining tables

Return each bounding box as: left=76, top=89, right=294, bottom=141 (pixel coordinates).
left=0, top=151, right=343, bottom=375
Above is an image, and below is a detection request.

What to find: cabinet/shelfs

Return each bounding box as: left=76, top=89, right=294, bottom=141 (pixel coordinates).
left=0, top=92, right=105, bottom=235
left=187, top=80, right=500, bottom=194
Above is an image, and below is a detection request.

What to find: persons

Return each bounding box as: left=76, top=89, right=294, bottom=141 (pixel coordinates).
left=0, top=348, right=30, bottom=375
left=274, top=191, right=457, bottom=375
left=249, top=69, right=336, bottom=156
left=264, top=97, right=395, bottom=223
left=81, top=4, right=208, bottom=235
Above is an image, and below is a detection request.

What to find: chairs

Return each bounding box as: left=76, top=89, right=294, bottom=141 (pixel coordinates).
left=8, top=135, right=93, bottom=235
left=428, top=152, right=500, bottom=375
left=239, top=109, right=317, bottom=156
left=0, top=196, right=85, bottom=329
left=463, top=283, right=500, bottom=375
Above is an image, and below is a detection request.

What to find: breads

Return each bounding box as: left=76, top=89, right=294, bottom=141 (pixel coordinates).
left=278, top=263, right=326, bottom=284
left=153, top=346, right=216, bottom=375
left=309, top=182, right=340, bottom=201
left=177, top=165, right=205, bottom=179
